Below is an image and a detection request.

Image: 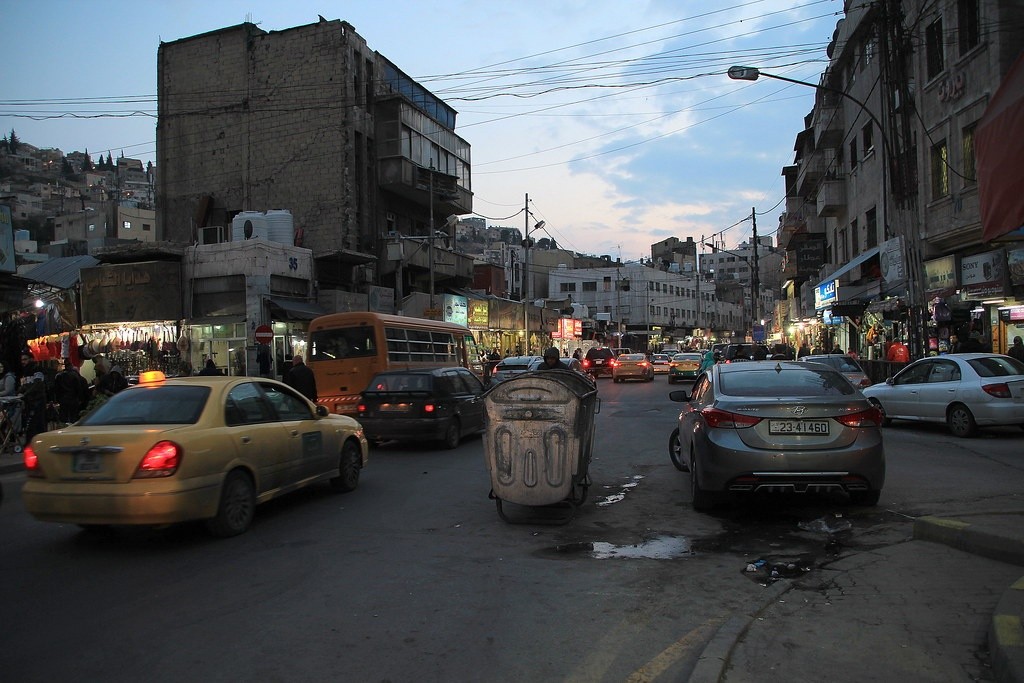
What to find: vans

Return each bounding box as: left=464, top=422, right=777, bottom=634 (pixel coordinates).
left=711, top=343, right=729, bottom=353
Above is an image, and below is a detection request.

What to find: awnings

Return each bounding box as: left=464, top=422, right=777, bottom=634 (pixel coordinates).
left=974, top=49, right=1024, bottom=242
left=813, top=247, right=880, bottom=289
left=263, top=296, right=328, bottom=318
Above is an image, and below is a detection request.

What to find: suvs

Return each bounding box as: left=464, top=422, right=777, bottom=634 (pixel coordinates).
left=583, top=346, right=619, bottom=377
left=720, top=342, right=771, bottom=363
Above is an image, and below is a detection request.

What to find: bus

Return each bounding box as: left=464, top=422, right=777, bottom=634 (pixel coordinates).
left=305, top=312, right=485, bottom=415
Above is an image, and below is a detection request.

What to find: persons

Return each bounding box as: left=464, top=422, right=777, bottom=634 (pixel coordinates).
left=830, top=345, right=844, bottom=354
left=684, top=344, right=690, bottom=352
left=848, top=348, right=856, bottom=358
left=46, top=359, right=88, bottom=424
left=1008, top=336, right=1024, bottom=364
left=491, top=348, right=500, bottom=359
left=92, top=355, right=127, bottom=396
left=732, top=343, right=766, bottom=360
left=282, top=355, right=318, bottom=404
left=199, top=359, right=222, bottom=376
left=770, top=343, right=810, bottom=361
left=701, top=349, right=721, bottom=370
left=573, top=350, right=579, bottom=360
left=812, top=346, right=822, bottom=354
left=947, top=334, right=964, bottom=353
left=44, top=360, right=61, bottom=385
left=651, top=343, right=661, bottom=353
left=885, top=336, right=909, bottom=362
left=536, top=347, right=568, bottom=370
left=0, top=351, right=48, bottom=447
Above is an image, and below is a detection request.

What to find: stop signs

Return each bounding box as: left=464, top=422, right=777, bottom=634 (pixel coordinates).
left=255, top=325, right=274, bottom=344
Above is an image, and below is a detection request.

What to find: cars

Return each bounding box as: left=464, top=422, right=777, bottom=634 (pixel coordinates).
left=862, top=353, right=1024, bottom=438
left=700, top=350, right=710, bottom=358
left=613, top=354, right=654, bottom=383
left=797, top=354, right=872, bottom=392
left=355, top=367, right=489, bottom=449
left=20, top=371, right=368, bottom=537
left=667, top=360, right=885, bottom=513
left=530, top=358, right=597, bottom=389
left=490, top=356, right=543, bottom=388
left=650, top=354, right=672, bottom=373
left=668, top=353, right=705, bottom=384
left=659, top=349, right=682, bottom=360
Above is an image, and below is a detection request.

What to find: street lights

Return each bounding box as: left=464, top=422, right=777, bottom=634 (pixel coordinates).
left=705, top=243, right=759, bottom=324
left=524, top=220, right=546, bottom=355
left=728, top=66, right=922, bottom=356
